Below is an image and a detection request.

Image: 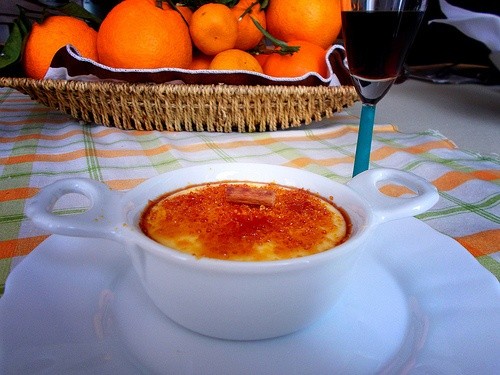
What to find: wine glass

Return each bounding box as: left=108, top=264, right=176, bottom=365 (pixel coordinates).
left=341, top=0, right=424, bottom=178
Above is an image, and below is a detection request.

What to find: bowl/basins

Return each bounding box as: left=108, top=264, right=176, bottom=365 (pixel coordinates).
left=25, top=165, right=440, bottom=341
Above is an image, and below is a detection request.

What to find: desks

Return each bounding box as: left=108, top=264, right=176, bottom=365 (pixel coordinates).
left=0, top=79, right=500, bottom=327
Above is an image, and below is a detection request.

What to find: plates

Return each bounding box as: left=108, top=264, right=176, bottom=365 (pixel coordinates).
left=0, top=216, right=500, bottom=375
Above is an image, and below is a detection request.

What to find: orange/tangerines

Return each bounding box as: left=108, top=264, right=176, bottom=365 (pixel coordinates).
left=23, top=0, right=353, bottom=81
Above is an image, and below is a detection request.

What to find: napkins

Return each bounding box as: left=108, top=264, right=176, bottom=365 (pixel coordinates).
left=428, top=0, right=500, bottom=52
